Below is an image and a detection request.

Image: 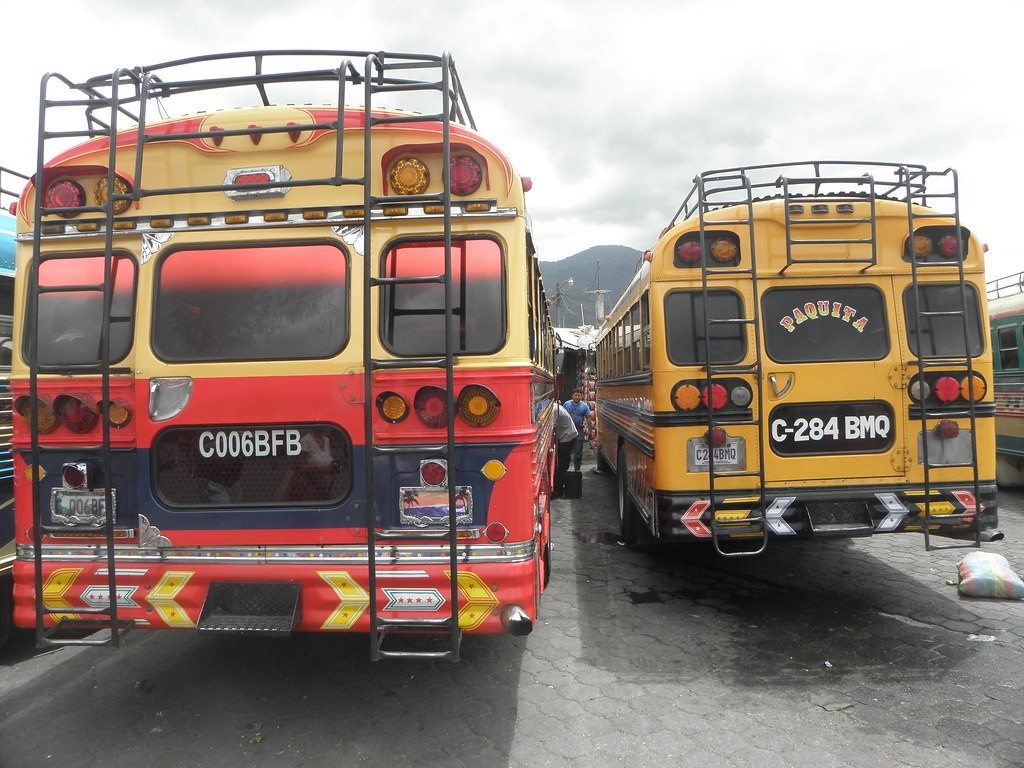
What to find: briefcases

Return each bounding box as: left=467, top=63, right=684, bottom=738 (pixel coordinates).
left=553, top=470, right=583, bottom=499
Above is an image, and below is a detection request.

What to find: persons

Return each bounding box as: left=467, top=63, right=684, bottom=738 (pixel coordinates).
left=563, top=388, right=592, bottom=472
left=550, top=402, right=578, bottom=500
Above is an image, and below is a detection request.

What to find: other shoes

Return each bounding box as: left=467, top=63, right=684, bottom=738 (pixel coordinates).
left=575, top=469, right=580, bottom=472
left=551, top=491, right=563, bottom=499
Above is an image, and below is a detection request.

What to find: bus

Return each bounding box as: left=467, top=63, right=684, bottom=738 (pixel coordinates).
left=582, top=159, right=1006, bottom=557
left=10, top=47, right=584, bottom=663
left=983, top=272, right=1024, bottom=460
left=0, top=166, right=30, bottom=576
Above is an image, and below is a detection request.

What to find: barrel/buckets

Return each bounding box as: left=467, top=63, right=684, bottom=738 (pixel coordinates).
left=563, top=471, right=582, bottom=499
